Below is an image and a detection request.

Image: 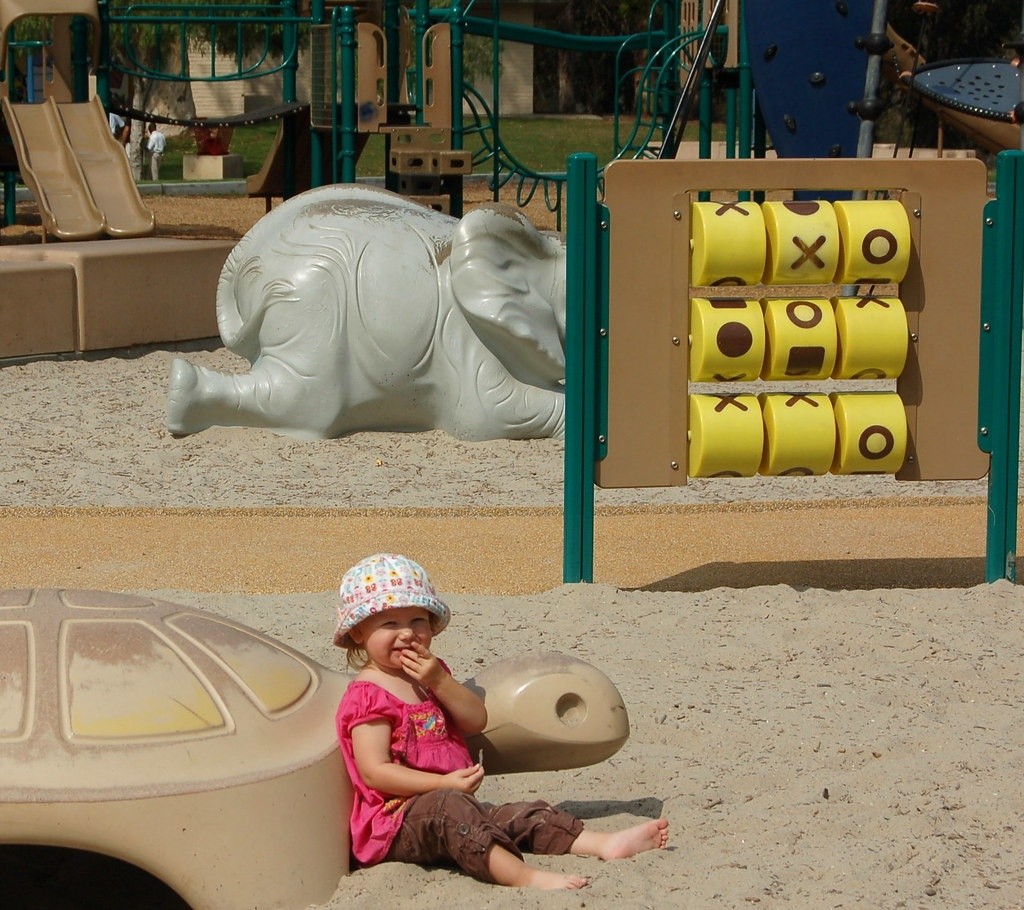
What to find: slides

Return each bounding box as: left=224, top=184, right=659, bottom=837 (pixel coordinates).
left=246, top=114, right=372, bottom=198
left=56, top=99, right=158, bottom=236
left=3, top=92, right=109, bottom=236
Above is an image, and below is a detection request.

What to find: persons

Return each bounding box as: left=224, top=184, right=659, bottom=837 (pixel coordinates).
left=109, top=112, right=125, bottom=136
left=334, top=553, right=670, bottom=890
left=1002, top=47, right=1020, bottom=123
left=120, top=117, right=131, bottom=160
left=145, top=122, right=166, bottom=181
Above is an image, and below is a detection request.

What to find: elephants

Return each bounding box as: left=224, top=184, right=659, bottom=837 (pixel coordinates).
left=166, top=183, right=569, bottom=443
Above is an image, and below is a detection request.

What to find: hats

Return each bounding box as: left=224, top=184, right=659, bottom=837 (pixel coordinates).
left=333, top=553, right=451, bottom=649
